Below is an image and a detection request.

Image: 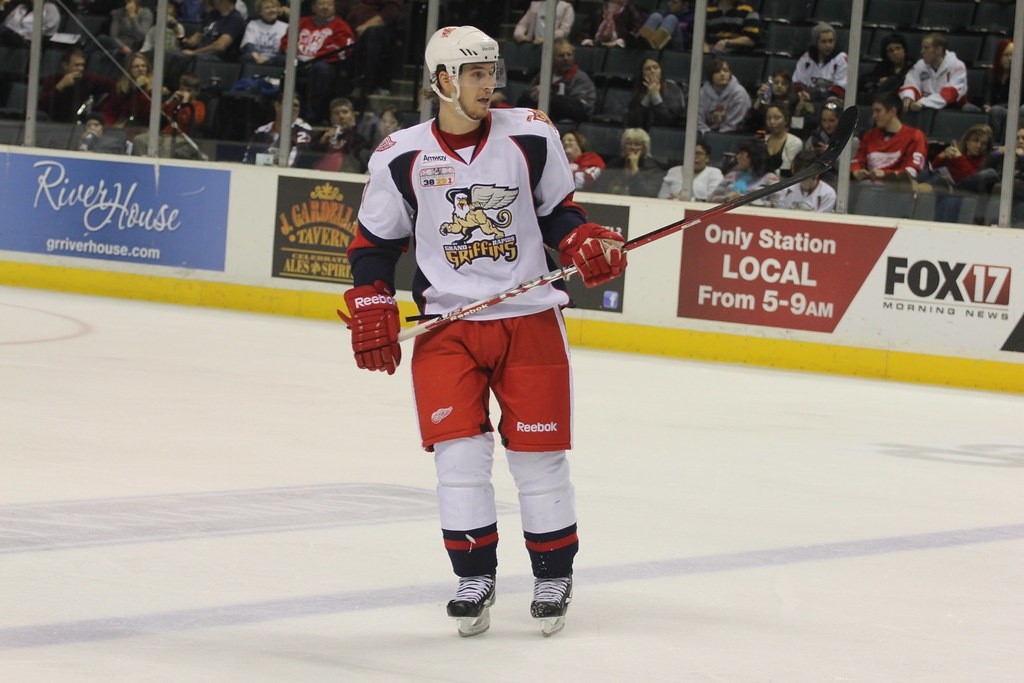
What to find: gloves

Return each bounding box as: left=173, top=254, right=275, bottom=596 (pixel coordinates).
left=338, top=281, right=404, bottom=375
left=559, top=222, right=630, bottom=287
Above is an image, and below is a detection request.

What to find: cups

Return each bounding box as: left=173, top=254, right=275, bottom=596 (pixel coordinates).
left=78, top=131, right=97, bottom=151
left=209, top=76, right=221, bottom=89
left=330, top=123, right=343, bottom=145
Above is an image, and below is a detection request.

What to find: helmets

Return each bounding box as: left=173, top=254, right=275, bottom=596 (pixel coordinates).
left=425, top=25, right=508, bottom=90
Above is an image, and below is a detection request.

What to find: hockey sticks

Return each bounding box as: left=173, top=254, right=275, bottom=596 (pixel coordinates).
left=399, top=105, right=860, bottom=341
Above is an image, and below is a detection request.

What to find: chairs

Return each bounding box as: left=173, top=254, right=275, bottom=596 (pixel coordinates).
left=0, top=1, right=1024, bottom=230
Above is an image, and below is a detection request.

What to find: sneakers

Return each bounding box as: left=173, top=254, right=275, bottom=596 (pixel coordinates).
left=446, top=574, right=500, bottom=635
left=529, top=576, right=577, bottom=637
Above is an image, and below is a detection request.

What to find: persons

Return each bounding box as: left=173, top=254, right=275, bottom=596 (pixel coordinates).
left=159, top=75, right=208, bottom=140
left=0, top=0, right=21, bottom=48
left=39, top=43, right=116, bottom=123
left=235, top=1, right=297, bottom=69
left=626, top=56, right=687, bottom=128
left=336, top=0, right=403, bottom=107
left=593, top=127, right=666, bottom=203
left=1, top=68, right=15, bottom=113
left=775, top=146, right=837, bottom=215
left=97, top=0, right=156, bottom=66
left=864, top=30, right=916, bottom=112
left=789, top=21, right=853, bottom=108
left=571, top=0, right=633, bottom=49
left=558, top=131, right=606, bottom=190
left=278, top=0, right=357, bottom=118
left=801, top=96, right=851, bottom=179
left=191, top=1, right=248, bottom=35
left=846, top=92, right=929, bottom=219
left=899, top=32, right=967, bottom=124
left=371, top=105, right=409, bottom=138
left=74, top=111, right=125, bottom=156
left=513, top=0, right=575, bottom=44
left=987, top=128, right=1023, bottom=205
left=734, top=72, right=805, bottom=133
left=241, top=90, right=315, bottom=170
left=487, top=87, right=513, bottom=108
left=622, top=0, right=693, bottom=52
left=755, top=100, right=804, bottom=178
left=137, top=1, right=187, bottom=59
left=307, top=96, right=378, bottom=173
left=120, top=50, right=155, bottom=125
left=987, top=39, right=1024, bottom=150
left=331, top=23, right=629, bottom=638
left=512, top=36, right=596, bottom=119
left=131, top=52, right=191, bottom=128
left=655, top=139, right=724, bottom=204
left=691, top=59, right=755, bottom=136
left=181, top=0, right=248, bottom=70
left=705, top=136, right=781, bottom=211
left=4, top=0, right=62, bottom=55
left=694, top=0, right=762, bottom=56
left=917, top=123, right=992, bottom=225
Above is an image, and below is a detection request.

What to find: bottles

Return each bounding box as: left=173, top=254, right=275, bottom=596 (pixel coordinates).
left=759, top=75, right=773, bottom=105
